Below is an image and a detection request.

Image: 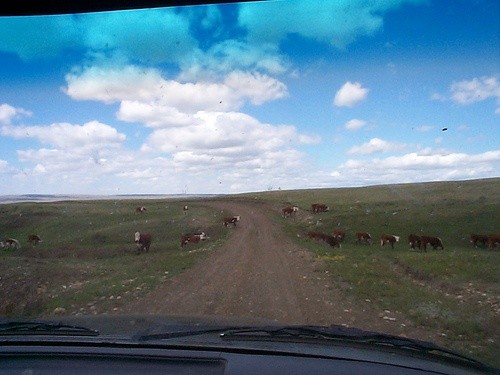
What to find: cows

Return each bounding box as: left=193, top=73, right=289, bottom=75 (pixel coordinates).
left=282, top=206, right=299, bottom=219
left=135, top=206, right=146, bottom=215
left=312, top=203, right=330, bottom=214
left=471, top=234, right=489, bottom=249
left=489, top=235, right=500, bottom=248
left=380, top=234, right=400, bottom=250
left=181, top=234, right=191, bottom=248
left=408, top=233, right=444, bottom=250
left=224, top=215, right=240, bottom=228
left=134, top=231, right=151, bottom=254
left=312, top=229, right=346, bottom=246
left=355, top=232, right=372, bottom=245
left=190, top=232, right=210, bottom=242
